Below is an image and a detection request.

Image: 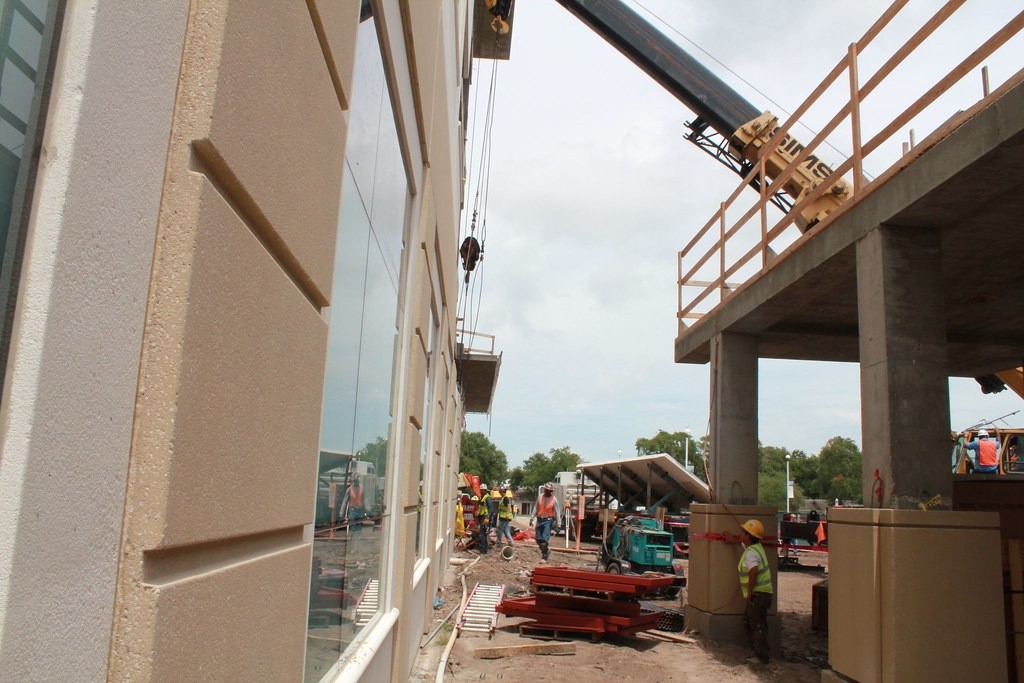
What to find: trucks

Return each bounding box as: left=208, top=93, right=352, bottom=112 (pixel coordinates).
left=536, top=468, right=696, bottom=559
left=314, top=458, right=386, bottom=526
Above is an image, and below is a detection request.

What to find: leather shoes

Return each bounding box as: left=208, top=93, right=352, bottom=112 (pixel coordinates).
left=539, top=548, right=551, bottom=564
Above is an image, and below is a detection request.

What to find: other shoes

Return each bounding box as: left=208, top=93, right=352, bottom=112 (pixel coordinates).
left=476, top=543, right=513, bottom=554
left=746, top=653, right=769, bottom=664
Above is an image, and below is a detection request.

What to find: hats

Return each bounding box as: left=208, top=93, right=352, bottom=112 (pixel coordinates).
left=497, top=486, right=508, bottom=491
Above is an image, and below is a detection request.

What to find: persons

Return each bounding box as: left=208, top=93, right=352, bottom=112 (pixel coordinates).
left=494, top=486, right=515, bottom=547
left=1006, top=444, right=1024, bottom=471
left=962, top=427, right=1002, bottom=474
left=781, top=513, right=800, bottom=557
left=529, top=481, right=563, bottom=565
left=735, top=518, right=776, bottom=664
left=476, top=483, right=494, bottom=552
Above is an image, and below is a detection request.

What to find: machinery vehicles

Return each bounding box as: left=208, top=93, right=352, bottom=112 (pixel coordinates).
left=601, top=514, right=685, bottom=599
left=450, top=1, right=1024, bottom=484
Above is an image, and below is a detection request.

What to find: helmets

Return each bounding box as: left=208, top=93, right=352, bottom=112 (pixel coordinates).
left=740, top=519, right=764, bottom=541
left=543, top=481, right=556, bottom=490
left=480, top=483, right=487, bottom=489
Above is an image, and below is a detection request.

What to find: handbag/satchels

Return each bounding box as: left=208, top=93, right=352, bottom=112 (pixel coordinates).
left=978, top=439, right=997, bottom=467
